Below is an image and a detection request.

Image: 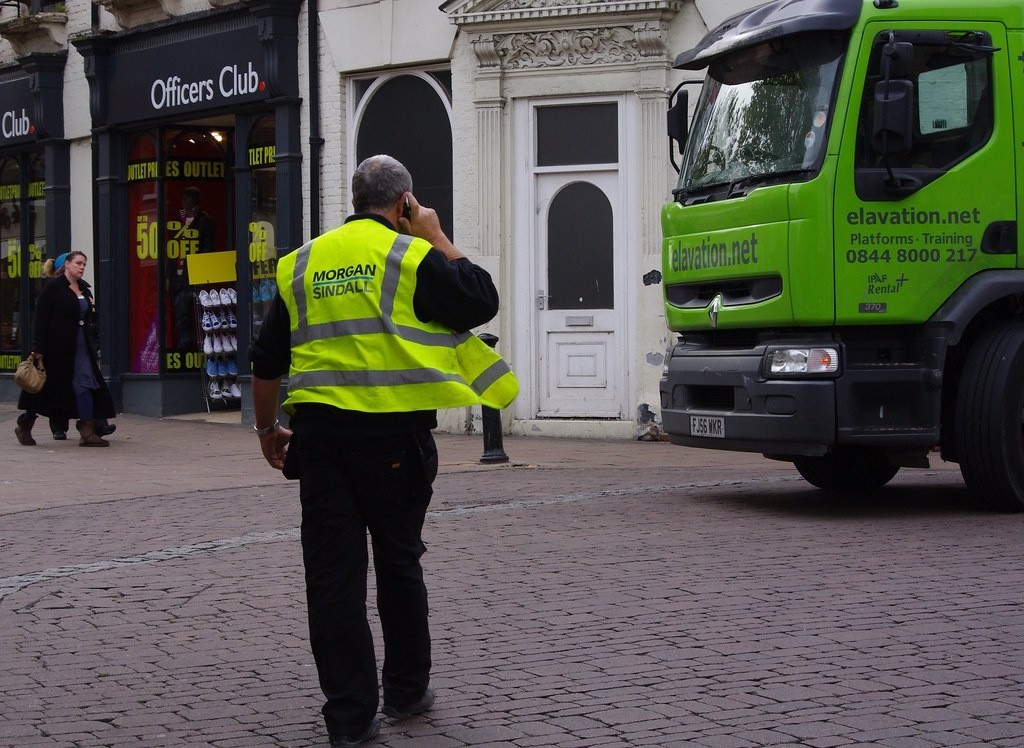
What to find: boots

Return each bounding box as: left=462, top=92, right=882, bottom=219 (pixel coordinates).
left=76, top=420, right=110, bottom=447
left=14, top=413, right=38, bottom=445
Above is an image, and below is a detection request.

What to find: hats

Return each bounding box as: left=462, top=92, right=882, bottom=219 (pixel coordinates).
left=53, top=249, right=74, bottom=270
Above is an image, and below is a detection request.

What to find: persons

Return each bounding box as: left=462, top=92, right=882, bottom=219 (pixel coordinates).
left=14, top=253, right=118, bottom=447
left=251, top=155, right=518, bottom=748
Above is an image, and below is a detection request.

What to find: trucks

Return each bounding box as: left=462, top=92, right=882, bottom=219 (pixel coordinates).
left=660, top=0, right=1024, bottom=512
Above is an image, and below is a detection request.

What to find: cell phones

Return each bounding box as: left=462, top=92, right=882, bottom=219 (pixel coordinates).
left=402, top=196, right=411, bottom=222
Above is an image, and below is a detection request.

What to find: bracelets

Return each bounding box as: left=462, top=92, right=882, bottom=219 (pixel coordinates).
left=253, top=419, right=279, bottom=435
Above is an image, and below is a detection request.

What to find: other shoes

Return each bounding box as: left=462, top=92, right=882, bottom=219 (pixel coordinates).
left=381, top=688, right=436, bottom=719
left=53, top=431, right=66, bottom=440
left=329, top=717, right=381, bottom=748
left=95, top=424, right=116, bottom=437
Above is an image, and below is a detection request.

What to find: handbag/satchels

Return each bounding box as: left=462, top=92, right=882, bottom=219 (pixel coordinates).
left=15, top=353, right=46, bottom=393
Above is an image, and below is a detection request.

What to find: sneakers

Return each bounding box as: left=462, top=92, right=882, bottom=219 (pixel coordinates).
left=219, top=288, right=231, bottom=306
left=210, top=289, right=221, bottom=307
left=209, top=310, right=221, bottom=330
left=226, top=309, right=237, bottom=328
left=225, top=357, right=238, bottom=375
left=206, top=356, right=218, bottom=377
left=253, top=311, right=263, bottom=325
left=252, top=287, right=261, bottom=303
left=266, top=280, right=277, bottom=301
left=217, top=309, right=229, bottom=329
left=229, top=333, right=238, bottom=351
left=199, top=290, right=213, bottom=307
left=210, top=380, right=241, bottom=398
left=259, top=281, right=271, bottom=302
left=217, top=355, right=227, bottom=376
left=201, top=309, right=213, bottom=331
left=227, top=288, right=238, bottom=305
left=203, top=333, right=213, bottom=354
left=221, top=331, right=233, bottom=352
left=213, top=333, right=222, bottom=353
